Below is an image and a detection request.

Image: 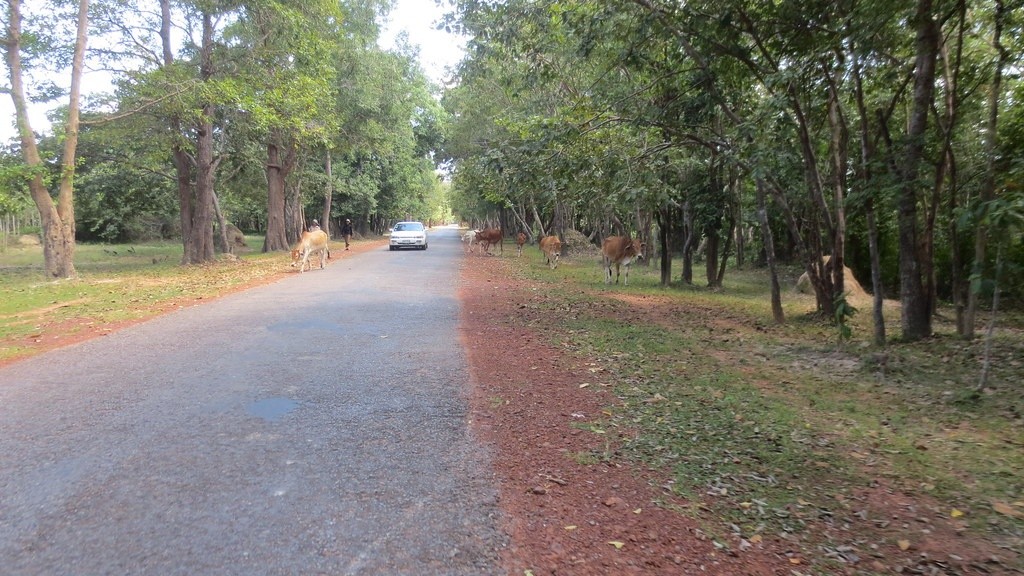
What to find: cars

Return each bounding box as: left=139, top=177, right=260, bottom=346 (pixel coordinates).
left=388, top=221, right=428, bottom=250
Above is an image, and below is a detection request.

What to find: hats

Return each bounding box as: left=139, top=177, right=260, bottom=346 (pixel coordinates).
left=313, top=219, right=319, bottom=226
left=345, top=219, right=350, bottom=222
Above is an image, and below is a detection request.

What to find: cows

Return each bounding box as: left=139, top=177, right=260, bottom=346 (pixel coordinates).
left=213, top=224, right=246, bottom=254
left=474, top=227, right=504, bottom=254
left=460, top=229, right=482, bottom=256
left=516, top=232, right=526, bottom=257
left=290, top=230, right=331, bottom=274
left=602, top=236, right=646, bottom=286
left=540, top=236, right=561, bottom=269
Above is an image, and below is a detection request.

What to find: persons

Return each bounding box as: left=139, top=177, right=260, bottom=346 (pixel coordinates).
left=309, top=219, right=321, bottom=231
left=341, top=219, right=352, bottom=250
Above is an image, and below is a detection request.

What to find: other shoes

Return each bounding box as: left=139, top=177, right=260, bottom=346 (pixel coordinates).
left=345, top=243, right=350, bottom=250
